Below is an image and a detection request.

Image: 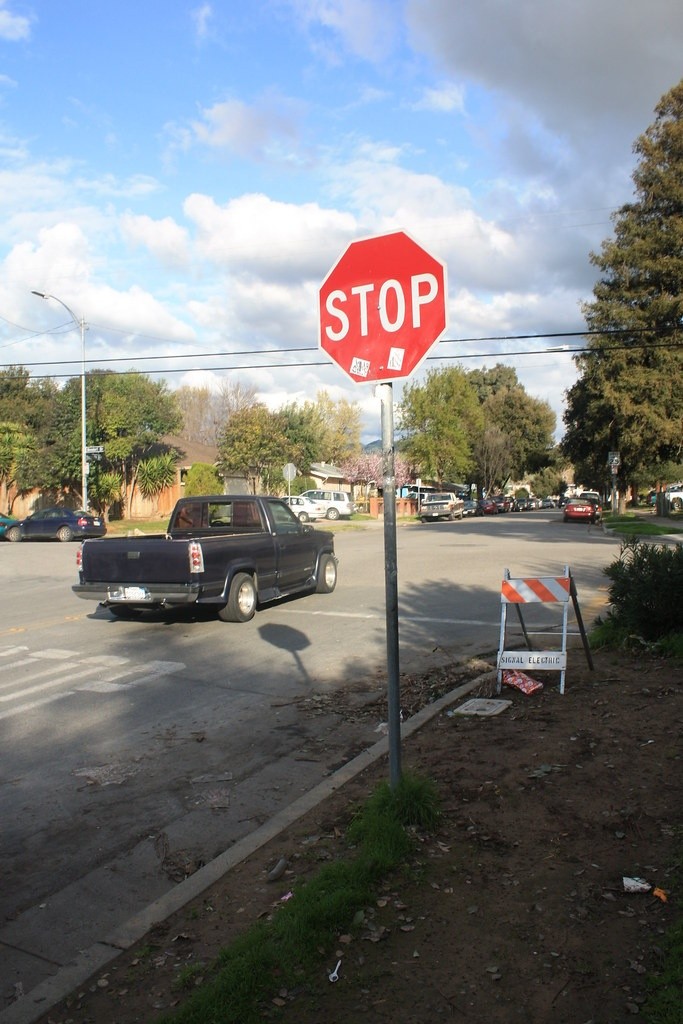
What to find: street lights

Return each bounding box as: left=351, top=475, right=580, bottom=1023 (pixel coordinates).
left=30, top=289, right=91, bottom=511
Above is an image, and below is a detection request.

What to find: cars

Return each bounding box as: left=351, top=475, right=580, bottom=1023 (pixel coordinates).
left=279, top=496, right=326, bottom=523
left=563, top=498, right=596, bottom=525
left=646, top=490, right=656, bottom=507
left=21, top=506, right=107, bottom=543
left=462, top=500, right=484, bottom=516
left=666, top=483, right=683, bottom=517
left=0, top=512, right=21, bottom=541
left=496, top=496, right=562, bottom=512
left=481, top=499, right=498, bottom=514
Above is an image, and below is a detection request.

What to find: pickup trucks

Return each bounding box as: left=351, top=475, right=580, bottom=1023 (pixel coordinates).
left=71, top=494, right=338, bottom=623
left=420, top=492, right=464, bottom=523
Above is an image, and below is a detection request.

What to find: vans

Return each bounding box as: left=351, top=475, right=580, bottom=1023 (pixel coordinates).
left=579, top=491, right=600, bottom=504
left=299, top=489, right=354, bottom=520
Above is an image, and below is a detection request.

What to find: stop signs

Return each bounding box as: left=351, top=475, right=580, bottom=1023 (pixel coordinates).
left=317, top=230, right=446, bottom=386
